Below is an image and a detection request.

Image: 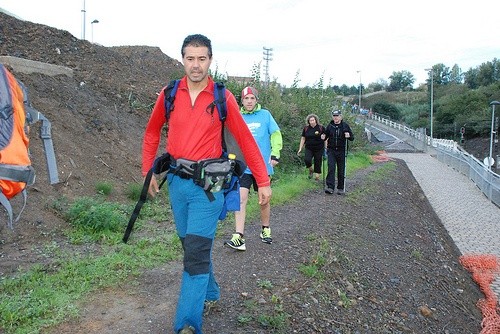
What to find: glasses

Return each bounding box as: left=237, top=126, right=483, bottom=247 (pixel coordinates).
left=333, top=115, right=338, bottom=116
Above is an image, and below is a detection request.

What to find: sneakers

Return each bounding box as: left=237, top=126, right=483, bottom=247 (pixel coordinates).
left=225, top=234, right=246, bottom=251
left=259, top=225, right=273, bottom=243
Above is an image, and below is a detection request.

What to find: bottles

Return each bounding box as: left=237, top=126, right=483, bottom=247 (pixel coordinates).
left=224, top=153, right=237, bottom=185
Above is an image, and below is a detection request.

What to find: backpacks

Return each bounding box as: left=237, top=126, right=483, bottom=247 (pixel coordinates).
left=0, top=63, right=60, bottom=229
left=163, top=78, right=248, bottom=177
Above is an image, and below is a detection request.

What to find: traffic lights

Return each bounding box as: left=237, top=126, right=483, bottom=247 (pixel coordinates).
left=461, top=139, right=465, bottom=144
left=461, top=127, right=465, bottom=134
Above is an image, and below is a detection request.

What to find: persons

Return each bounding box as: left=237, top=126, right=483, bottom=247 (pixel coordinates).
left=321, top=110, right=354, bottom=195
left=224, top=86, right=283, bottom=250
left=343, top=101, right=373, bottom=119
left=142, top=35, right=272, bottom=334
left=297, top=114, right=325, bottom=182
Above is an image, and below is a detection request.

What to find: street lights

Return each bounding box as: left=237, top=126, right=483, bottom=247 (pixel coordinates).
left=489, top=100, right=500, bottom=171
left=91, top=19, right=99, bottom=42
left=81, top=10, right=87, bottom=39
left=356, top=70, right=361, bottom=109
left=424, top=68, right=433, bottom=139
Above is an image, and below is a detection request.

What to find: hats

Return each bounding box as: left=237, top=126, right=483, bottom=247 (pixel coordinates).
left=332, top=110, right=341, bottom=115
left=241, top=86, right=259, bottom=100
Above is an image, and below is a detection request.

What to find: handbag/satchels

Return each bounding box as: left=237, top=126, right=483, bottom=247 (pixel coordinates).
left=192, top=156, right=237, bottom=200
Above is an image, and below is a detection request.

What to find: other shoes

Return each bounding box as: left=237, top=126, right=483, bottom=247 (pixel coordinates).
left=203, top=299, right=218, bottom=316
left=180, top=324, right=196, bottom=334
left=337, top=188, right=344, bottom=195
left=325, top=188, right=334, bottom=194
left=308, top=169, right=313, bottom=178
